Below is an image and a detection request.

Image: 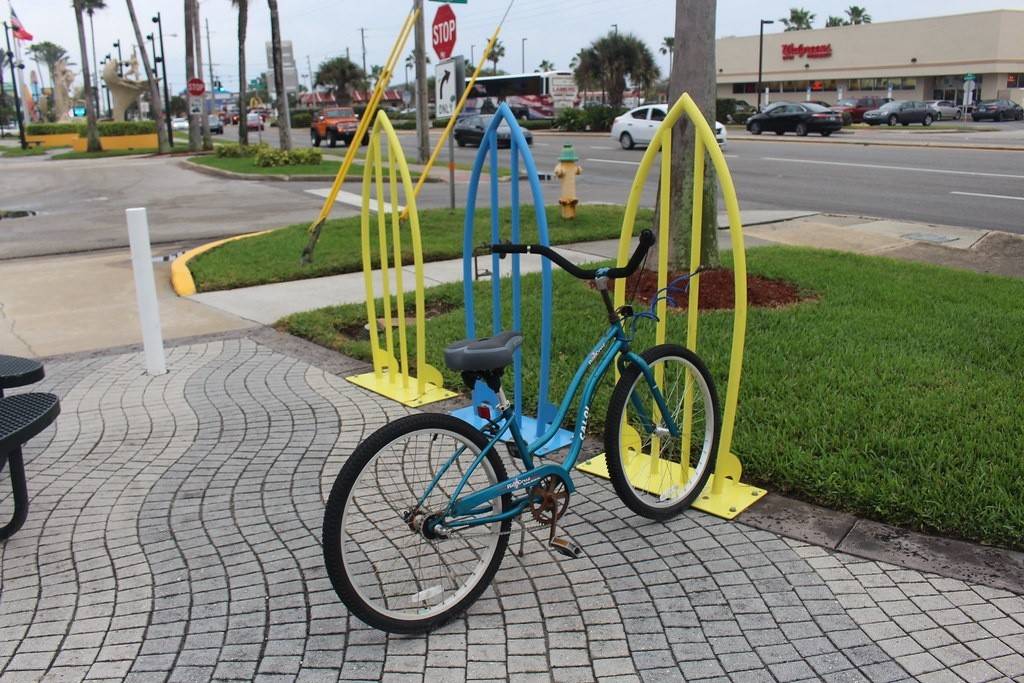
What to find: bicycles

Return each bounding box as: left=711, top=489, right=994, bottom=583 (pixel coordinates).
left=318, top=226, right=722, bottom=635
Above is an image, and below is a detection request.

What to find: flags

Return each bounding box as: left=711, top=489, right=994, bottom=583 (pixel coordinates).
left=11, top=5, right=33, bottom=41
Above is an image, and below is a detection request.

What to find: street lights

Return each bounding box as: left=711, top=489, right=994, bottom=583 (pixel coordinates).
left=99, top=60, right=112, bottom=118
left=2, top=21, right=29, bottom=149
left=756, top=18, right=775, bottom=114
left=133, top=33, right=178, bottom=121
left=146, top=33, right=159, bottom=91
left=113, top=38, right=129, bottom=79
left=522, top=37, right=528, bottom=73
left=470, top=44, right=476, bottom=74
left=152, top=11, right=174, bottom=147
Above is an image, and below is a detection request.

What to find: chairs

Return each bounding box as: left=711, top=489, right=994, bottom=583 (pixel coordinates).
left=0, top=353, right=60, bottom=537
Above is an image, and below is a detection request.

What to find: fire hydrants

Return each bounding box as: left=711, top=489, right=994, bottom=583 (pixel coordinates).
left=553, top=142, right=584, bottom=220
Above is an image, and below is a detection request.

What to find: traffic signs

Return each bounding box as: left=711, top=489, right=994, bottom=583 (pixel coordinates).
left=434, top=59, right=458, bottom=118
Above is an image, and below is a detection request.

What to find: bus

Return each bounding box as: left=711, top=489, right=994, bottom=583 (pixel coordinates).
left=464, top=71, right=583, bottom=122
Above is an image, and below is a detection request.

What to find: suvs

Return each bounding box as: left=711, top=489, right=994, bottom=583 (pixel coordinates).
left=309, top=106, right=370, bottom=148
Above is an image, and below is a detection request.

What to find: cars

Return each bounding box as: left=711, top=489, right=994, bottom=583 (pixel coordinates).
left=585, top=100, right=603, bottom=107
left=452, top=113, right=534, bottom=150
left=799, top=95, right=896, bottom=126
left=609, top=102, right=729, bottom=151
left=862, top=99, right=939, bottom=127
left=971, top=98, right=1024, bottom=123
left=744, top=101, right=844, bottom=137
left=168, top=108, right=273, bottom=135
left=918, top=98, right=962, bottom=122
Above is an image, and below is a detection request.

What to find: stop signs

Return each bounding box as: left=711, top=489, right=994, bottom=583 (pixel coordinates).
left=188, top=78, right=206, bottom=96
left=431, top=1, right=457, bottom=60
